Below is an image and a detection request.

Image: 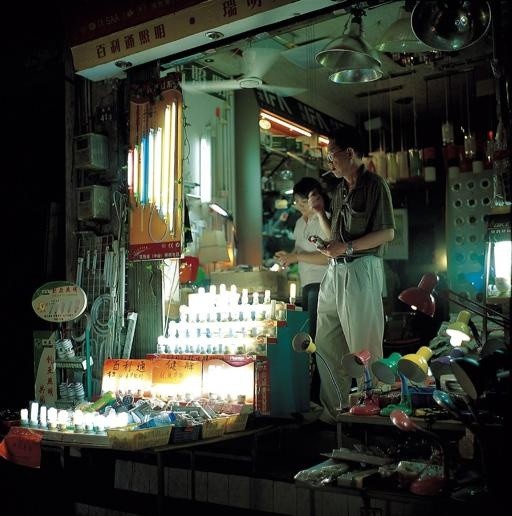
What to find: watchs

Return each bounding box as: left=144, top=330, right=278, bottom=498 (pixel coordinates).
left=346, top=240, right=353, bottom=256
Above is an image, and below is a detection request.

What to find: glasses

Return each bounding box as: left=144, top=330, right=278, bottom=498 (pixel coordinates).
left=327, top=147, right=347, bottom=161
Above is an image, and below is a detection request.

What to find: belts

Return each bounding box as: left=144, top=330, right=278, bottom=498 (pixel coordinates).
left=329, top=256, right=361, bottom=265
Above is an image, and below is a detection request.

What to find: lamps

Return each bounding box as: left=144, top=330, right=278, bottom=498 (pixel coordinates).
left=307, top=0, right=512, bottom=190
left=290, top=272, right=512, bottom=507
left=124, top=93, right=183, bottom=250
left=19, top=284, right=283, bottom=452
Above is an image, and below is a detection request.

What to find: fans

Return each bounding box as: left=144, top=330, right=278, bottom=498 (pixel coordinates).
left=174, top=37, right=307, bottom=102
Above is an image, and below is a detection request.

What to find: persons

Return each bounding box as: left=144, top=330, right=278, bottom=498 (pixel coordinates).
left=309, top=123, right=397, bottom=423
left=274, top=176, right=332, bottom=342
